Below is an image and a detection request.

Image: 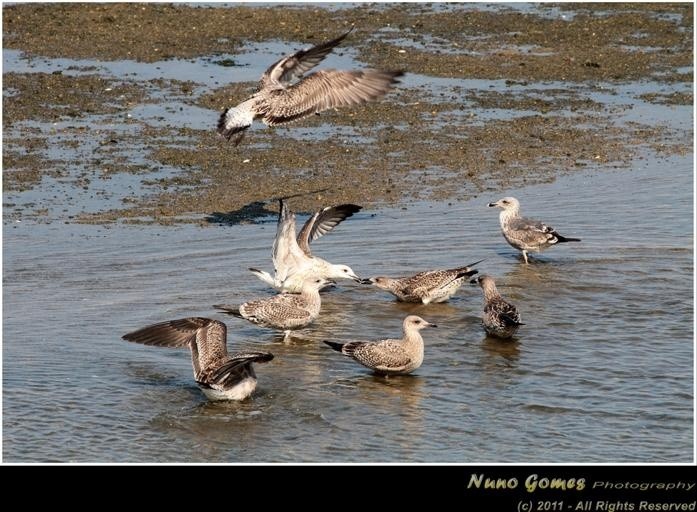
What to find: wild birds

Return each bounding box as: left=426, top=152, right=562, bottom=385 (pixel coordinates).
left=361, top=258, right=486, bottom=305
left=469, top=274, right=527, bottom=338
left=488, top=196, right=582, bottom=265
left=323, top=315, right=438, bottom=379
left=211, top=275, right=337, bottom=343
left=120, top=316, right=275, bottom=403
left=247, top=198, right=364, bottom=295
left=216, top=24, right=408, bottom=150
